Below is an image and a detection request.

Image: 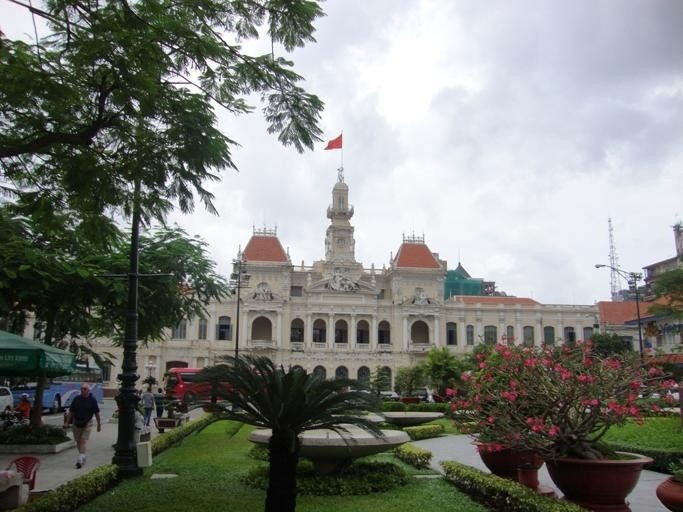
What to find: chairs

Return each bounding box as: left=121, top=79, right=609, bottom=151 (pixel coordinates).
left=4, top=456, right=40, bottom=495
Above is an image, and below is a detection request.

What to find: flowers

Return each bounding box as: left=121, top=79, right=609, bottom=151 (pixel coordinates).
left=446, top=337, right=677, bottom=459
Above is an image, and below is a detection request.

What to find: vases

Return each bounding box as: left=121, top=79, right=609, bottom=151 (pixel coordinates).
left=476, top=441, right=552, bottom=491
left=546, top=451, right=649, bottom=512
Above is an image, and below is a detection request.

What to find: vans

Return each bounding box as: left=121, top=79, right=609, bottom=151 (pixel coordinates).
left=0, top=386, right=14, bottom=416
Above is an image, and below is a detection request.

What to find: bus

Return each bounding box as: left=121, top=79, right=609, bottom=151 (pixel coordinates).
left=163, top=368, right=268, bottom=400
left=11, top=367, right=104, bottom=413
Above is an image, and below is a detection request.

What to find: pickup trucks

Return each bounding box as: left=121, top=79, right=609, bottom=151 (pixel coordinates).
left=402, top=387, right=431, bottom=403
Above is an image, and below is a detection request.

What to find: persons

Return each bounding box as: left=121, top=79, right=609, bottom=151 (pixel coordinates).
left=62, top=383, right=102, bottom=469
left=152, top=387, right=166, bottom=418
left=13, top=393, right=31, bottom=419
left=141, top=386, right=154, bottom=426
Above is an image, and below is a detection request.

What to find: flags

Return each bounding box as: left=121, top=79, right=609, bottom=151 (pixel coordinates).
left=323, top=134, right=343, bottom=151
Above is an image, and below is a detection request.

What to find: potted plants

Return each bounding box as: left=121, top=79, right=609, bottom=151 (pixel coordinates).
left=656, top=458, right=683, bottom=512
left=425, top=344, right=461, bottom=403
left=393, top=365, right=423, bottom=405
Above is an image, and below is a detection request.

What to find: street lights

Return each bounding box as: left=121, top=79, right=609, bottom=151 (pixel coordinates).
left=227, top=245, right=252, bottom=412
left=595, top=265, right=643, bottom=364
left=145, top=361, right=159, bottom=388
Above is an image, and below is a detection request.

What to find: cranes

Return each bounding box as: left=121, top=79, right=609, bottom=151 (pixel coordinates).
left=608, top=217, right=622, bottom=300
